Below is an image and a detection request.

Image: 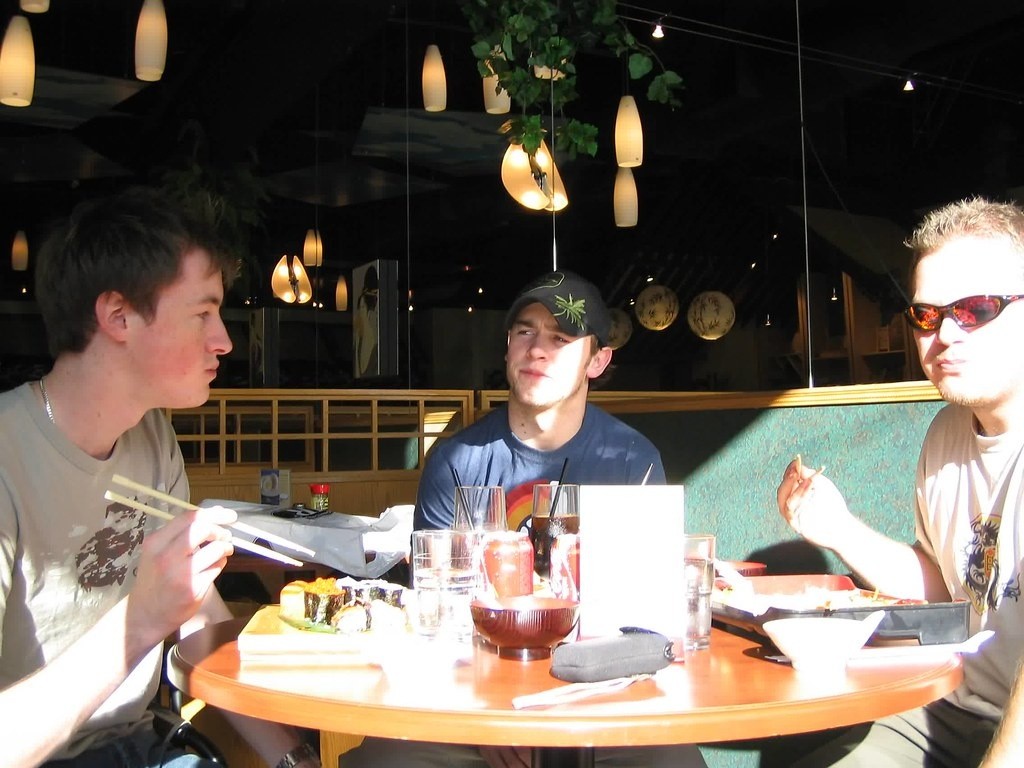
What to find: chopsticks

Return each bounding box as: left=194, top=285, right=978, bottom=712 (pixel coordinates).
left=795, top=453, right=826, bottom=481
left=101, top=474, right=318, bottom=566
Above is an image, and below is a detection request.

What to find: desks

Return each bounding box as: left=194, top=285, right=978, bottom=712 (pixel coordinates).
left=189, top=573, right=1023, bottom=767
left=223, top=556, right=407, bottom=606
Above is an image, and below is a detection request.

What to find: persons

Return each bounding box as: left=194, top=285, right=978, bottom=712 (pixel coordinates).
left=344, top=271, right=710, bottom=768
left=0, top=194, right=325, bottom=768
left=776, top=198, right=1024, bottom=768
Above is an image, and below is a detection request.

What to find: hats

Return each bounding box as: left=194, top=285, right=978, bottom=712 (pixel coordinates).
left=505, top=272, right=611, bottom=349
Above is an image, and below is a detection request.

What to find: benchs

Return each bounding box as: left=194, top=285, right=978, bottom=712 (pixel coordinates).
left=607, top=402, right=1022, bottom=735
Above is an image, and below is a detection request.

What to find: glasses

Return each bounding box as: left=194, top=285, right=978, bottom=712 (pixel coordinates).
left=902, top=294, right=1024, bottom=330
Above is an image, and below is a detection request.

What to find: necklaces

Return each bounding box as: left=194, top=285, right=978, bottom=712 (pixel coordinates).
left=39, top=375, right=56, bottom=426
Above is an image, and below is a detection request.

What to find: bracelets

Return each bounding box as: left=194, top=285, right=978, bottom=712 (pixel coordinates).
left=277, top=741, right=319, bottom=768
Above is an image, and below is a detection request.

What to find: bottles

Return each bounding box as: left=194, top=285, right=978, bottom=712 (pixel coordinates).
left=308, top=483, right=329, bottom=512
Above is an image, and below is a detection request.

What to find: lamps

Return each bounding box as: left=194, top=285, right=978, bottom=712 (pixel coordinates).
left=603, top=307, right=633, bottom=348
left=690, top=290, right=739, bottom=342
left=0, top=0, right=50, bottom=108
left=135, top=0, right=168, bottom=81
left=334, top=272, right=349, bottom=309
left=424, top=24, right=644, bottom=230
left=272, top=227, right=325, bottom=305
left=11, top=229, right=28, bottom=271
left=629, top=286, right=680, bottom=331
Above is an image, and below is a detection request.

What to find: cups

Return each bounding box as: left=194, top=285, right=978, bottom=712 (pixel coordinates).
left=682, top=533, right=717, bottom=650
left=411, top=528, right=478, bottom=637
left=530, top=483, right=580, bottom=583
left=453, top=486, right=509, bottom=532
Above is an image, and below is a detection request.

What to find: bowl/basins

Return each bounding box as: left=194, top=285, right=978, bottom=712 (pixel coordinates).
left=469, top=596, right=580, bottom=663
left=762, top=617, right=866, bottom=673
left=716, top=560, right=769, bottom=577
